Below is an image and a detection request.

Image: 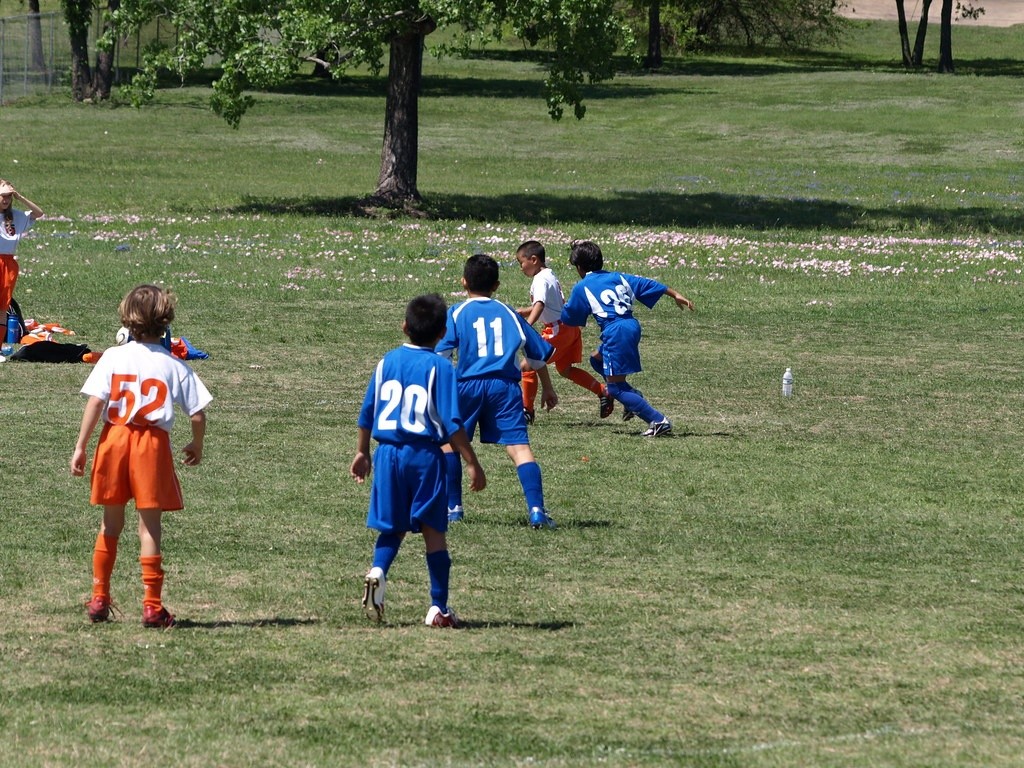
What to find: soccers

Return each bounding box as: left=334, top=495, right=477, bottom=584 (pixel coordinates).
left=115, top=325, right=130, bottom=346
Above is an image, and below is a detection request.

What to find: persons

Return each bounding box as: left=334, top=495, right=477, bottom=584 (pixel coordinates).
left=515, top=241, right=614, bottom=422
left=71, top=286, right=214, bottom=625
left=559, top=241, right=694, bottom=438
left=435, top=254, right=558, bottom=527
left=0, top=179, right=43, bottom=363
left=350, top=292, right=488, bottom=628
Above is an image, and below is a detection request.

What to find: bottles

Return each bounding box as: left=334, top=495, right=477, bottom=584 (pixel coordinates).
left=783, top=368, right=793, bottom=397
left=8, top=312, right=19, bottom=343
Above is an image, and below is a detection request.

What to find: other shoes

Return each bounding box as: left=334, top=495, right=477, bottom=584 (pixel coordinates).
left=0, top=353, right=6, bottom=362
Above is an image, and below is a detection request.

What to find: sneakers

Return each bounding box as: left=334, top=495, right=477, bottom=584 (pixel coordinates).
left=141, top=605, right=175, bottom=627
left=424, top=606, right=458, bottom=628
left=85, top=597, right=124, bottom=621
left=599, top=386, right=614, bottom=418
left=621, top=389, right=645, bottom=421
left=447, top=505, right=463, bottom=521
left=642, top=416, right=671, bottom=437
left=523, top=407, right=534, bottom=424
left=529, top=507, right=556, bottom=529
left=361, top=567, right=386, bottom=621
